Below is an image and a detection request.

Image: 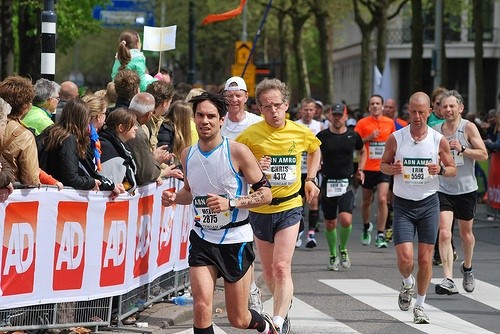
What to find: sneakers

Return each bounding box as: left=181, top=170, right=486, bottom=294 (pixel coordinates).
left=260, top=312, right=278, bottom=334
left=339, top=249, right=351, bottom=268
left=306, top=234, right=317, bottom=248
left=460, top=260, right=475, bottom=292
left=385, top=229, right=393, bottom=243
left=375, top=233, right=388, bottom=248
left=328, top=255, right=340, bottom=270
left=277, top=314, right=291, bottom=334
left=453, top=249, right=458, bottom=261
left=360, top=222, right=373, bottom=246
left=413, top=306, right=430, bottom=324
left=436, top=278, right=459, bottom=294
left=433, top=250, right=442, bottom=265
left=398, top=275, right=416, bottom=310
left=295, top=230, right=304, bottom=247
left=249, top=287, right=263, bottom=315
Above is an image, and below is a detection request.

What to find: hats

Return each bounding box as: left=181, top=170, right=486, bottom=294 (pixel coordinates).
left=315, top=101, right=323, bottom=109
left=224, top=76, right=248, bottom=91
left=330, top=104, right=348, bottom=115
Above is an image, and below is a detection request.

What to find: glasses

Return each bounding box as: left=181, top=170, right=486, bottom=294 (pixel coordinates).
left=47, top=96, right=61, bottom=101
left=102, top=110, right=110, bottom=114
left=258, top=101, right=284, bottom=111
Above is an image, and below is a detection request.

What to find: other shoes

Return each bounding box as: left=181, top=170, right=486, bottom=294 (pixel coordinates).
left=112, top=317, right=136, bottom=324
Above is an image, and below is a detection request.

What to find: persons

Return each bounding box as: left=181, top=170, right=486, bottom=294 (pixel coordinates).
left=233, top=80, right=322, bottom=334
left=426, top=88, right=458, bottom=266
left=290, top=97, right=327, bottom=249
left=313, top=103, right=366, bottom=271
left=111, top=30, right=159, bottom=92
left=158, top=92, right=280, bottom=334
left=347, top=94, right=409, bottom=249
left=466, top=108, right=500, bottom=222
left=379, top=92, right=458, bottom=325
left=429, top=90, right=489, bottom=296
left=216, top=76, right=265, bottom=315
left=0, top=67, right=263, bottom=334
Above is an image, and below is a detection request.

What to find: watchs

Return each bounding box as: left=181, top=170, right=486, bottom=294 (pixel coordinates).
left=438, top=166, right=446, bottom=175
left=461, top=145, right=466, bottom=153
left=305, top=177, right=318, bottom=186
left=229, top=198, right=236, bottom=211
